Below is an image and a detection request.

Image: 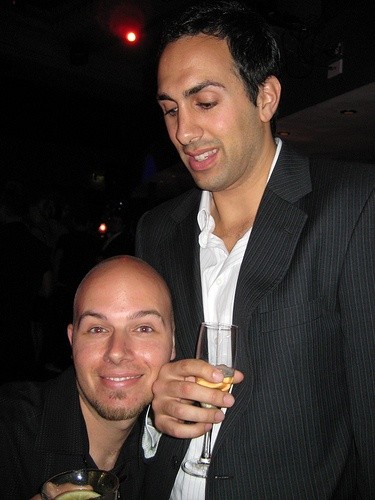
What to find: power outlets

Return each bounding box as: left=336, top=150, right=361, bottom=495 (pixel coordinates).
left=327, top=58, right=343, bottom=79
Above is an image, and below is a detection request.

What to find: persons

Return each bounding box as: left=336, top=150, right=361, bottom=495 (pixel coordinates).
left=0, top=255, right=176, bottom=500
left=135, top=0, right=375, bottom=500
left=0, top=180, right=137, bottom=382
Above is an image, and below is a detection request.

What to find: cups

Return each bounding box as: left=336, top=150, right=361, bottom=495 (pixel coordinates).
left=40, top=469, right=119, bottom=500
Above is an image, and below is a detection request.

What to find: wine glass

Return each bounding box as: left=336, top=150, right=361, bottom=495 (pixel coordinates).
left=183, top=322, right=238, bottom=478
